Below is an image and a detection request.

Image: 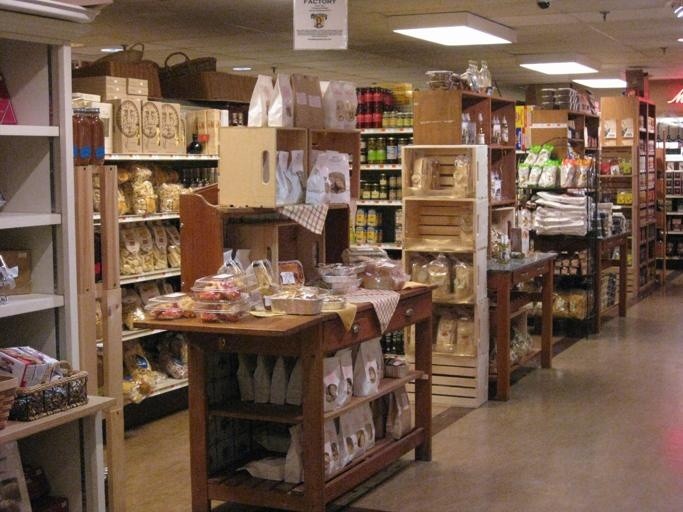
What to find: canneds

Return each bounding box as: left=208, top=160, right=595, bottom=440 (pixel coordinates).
left=350, top=86, right=414, bottom=247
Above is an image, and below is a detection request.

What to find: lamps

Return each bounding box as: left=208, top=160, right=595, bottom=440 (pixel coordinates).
left=514, top=52, right=600, bottom=78
left=387, top=12, right=518, bottom=49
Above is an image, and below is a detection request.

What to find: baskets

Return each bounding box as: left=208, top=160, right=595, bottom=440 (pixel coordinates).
left=83, top=43, right=257, bottom=103
left=0, top=360, right=87, bottom=429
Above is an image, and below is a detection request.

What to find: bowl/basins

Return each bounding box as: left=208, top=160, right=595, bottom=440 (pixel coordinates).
left=322, top=275, right=362, bottom=292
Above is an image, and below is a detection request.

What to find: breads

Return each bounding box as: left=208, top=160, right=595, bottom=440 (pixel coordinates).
left=454, top=164, right=474, bottom=198
left=408, top=262, right=475, bottom=354
left=412, top=157, right=439, bottom=190
left=460, top=213, right=474, bottom=247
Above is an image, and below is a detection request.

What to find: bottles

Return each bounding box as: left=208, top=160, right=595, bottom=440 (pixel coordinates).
left=181, top=168, right=219, bottom=189
left=461, top=60, right=519, bottom=263
left=350, top=84, right=414, bottom=250
left=381, top=327, right=405, bottom=354
left=230, top=112, right=243, bottom=126
left=72, top=107, right=106, bottom=159
left=188, top=134, right=203, bottom=154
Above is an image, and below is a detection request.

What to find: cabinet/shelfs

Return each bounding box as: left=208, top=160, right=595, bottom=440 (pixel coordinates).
left=79, top=90, right=683, bottom=398
left=0, top=398, right=118, bottom=511
left=0, top=0, right=116, bottom=394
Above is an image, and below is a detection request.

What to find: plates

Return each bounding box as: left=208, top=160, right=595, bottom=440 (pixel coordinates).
left=318, top=296, right=343, bottom=310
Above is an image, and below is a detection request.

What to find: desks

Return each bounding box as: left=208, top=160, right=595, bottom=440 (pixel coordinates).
left=132, top=272, right=437, bottom=512
